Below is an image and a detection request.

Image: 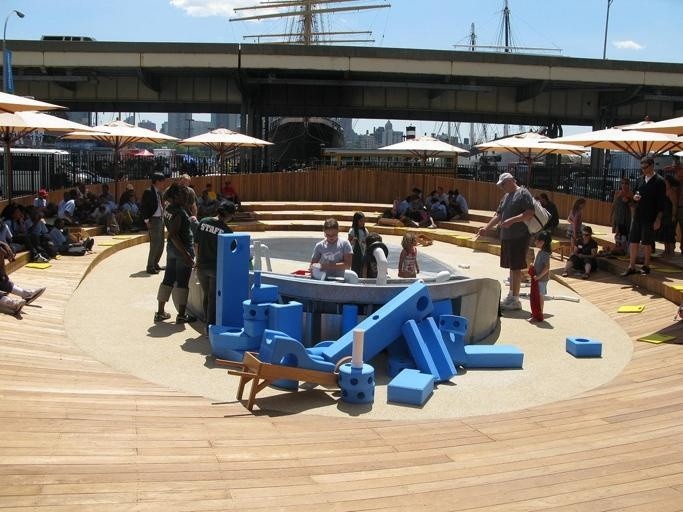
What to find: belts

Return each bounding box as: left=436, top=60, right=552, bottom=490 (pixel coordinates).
left=150, top=217, right=162, bottom=219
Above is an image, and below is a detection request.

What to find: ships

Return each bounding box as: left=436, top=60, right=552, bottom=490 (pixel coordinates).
left=228, top=0, right=393, bottom=173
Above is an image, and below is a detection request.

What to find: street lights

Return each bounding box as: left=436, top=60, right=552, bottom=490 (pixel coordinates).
left=1, top=8, right=26, bottom=95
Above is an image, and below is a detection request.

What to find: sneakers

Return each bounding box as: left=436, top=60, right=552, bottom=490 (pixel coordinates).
left=620, top=268, right=636, bottom=276
left=176, top=314, right=197, bottom=323
left=34, top=254, right=48, bottom=263
left=582, top=273, right=590, bottom=279
left=500, top=297, right=522, bottom=310
left=528, top=317, right=538, bottom=322
left=155, top=312, right=171, bottom=321
left=640, top=265, right=650, bottom=273
left=83, top=237, right=94, bottom=250
left=427, top=224, right=437, bottom=228
left=22, top=288, right=46, bottom=305
left=9, top=299, right=25, bottom=313
left=562, top=271, right=569, bottom=276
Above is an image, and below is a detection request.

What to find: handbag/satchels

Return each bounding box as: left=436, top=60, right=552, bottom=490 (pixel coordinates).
left=523, top=197, right=552, bottom=233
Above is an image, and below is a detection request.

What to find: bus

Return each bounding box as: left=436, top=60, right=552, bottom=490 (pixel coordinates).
left=0, top=146, right=74, bottom=195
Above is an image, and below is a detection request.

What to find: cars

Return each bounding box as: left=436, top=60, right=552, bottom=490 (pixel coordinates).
left=457, top=152, right=640, bottom=205
left=74, top=168, right=115, bottom=185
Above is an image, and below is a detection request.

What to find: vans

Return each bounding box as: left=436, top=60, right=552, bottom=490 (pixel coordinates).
left=39, top=34, right=96, bottom=43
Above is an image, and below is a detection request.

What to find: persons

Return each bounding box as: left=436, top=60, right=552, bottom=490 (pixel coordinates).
left=397, top=232, right=419, bottom=278
left=0, top=172, right=241, bottom=325
left=526, top=230, right=552, bottom=323
left=308, top=218, right=353, bottom=280
left=533, top=155, right=682, bottom=278
left=476, top=172, right=535, bottom=312
left=362, top=232, right=388, bottom=278
left=347, top=211, right=369, bottom=272
left=379, top=186, right=470, bottom=231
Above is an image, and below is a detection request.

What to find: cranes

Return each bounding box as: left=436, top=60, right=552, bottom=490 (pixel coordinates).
left=434, top=0, right=562, bottom=171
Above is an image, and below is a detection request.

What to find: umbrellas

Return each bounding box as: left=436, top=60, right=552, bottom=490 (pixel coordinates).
left=474, top=129, right=593, bottom=193
left=0, top=92, right=68, bottom=114
left=0, top=111, right=110, bottom=196
left=376, top=133, right=471, bottom=203
left=537, top=115, right=683, bottom=165
left=176, top=126, right=277, bottom=195
left=618, top=114, right=683, bottom=136
left=60, top=119, right=182, bottom=204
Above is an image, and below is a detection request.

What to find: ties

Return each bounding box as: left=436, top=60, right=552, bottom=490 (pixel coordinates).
left=158, top=192, right=163, bottom=218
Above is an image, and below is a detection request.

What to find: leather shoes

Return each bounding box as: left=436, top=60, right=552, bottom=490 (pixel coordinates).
left=147, top=265, right=164, bottom=274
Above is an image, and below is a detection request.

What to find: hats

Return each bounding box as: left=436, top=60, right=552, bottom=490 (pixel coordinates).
left=220, top=201, right=236, bottom=216
left=496, top=173, right=514, bottom=185
left=126, top=184, right=135, bottom=190
left=39, top=190, right=48, bottom=197
